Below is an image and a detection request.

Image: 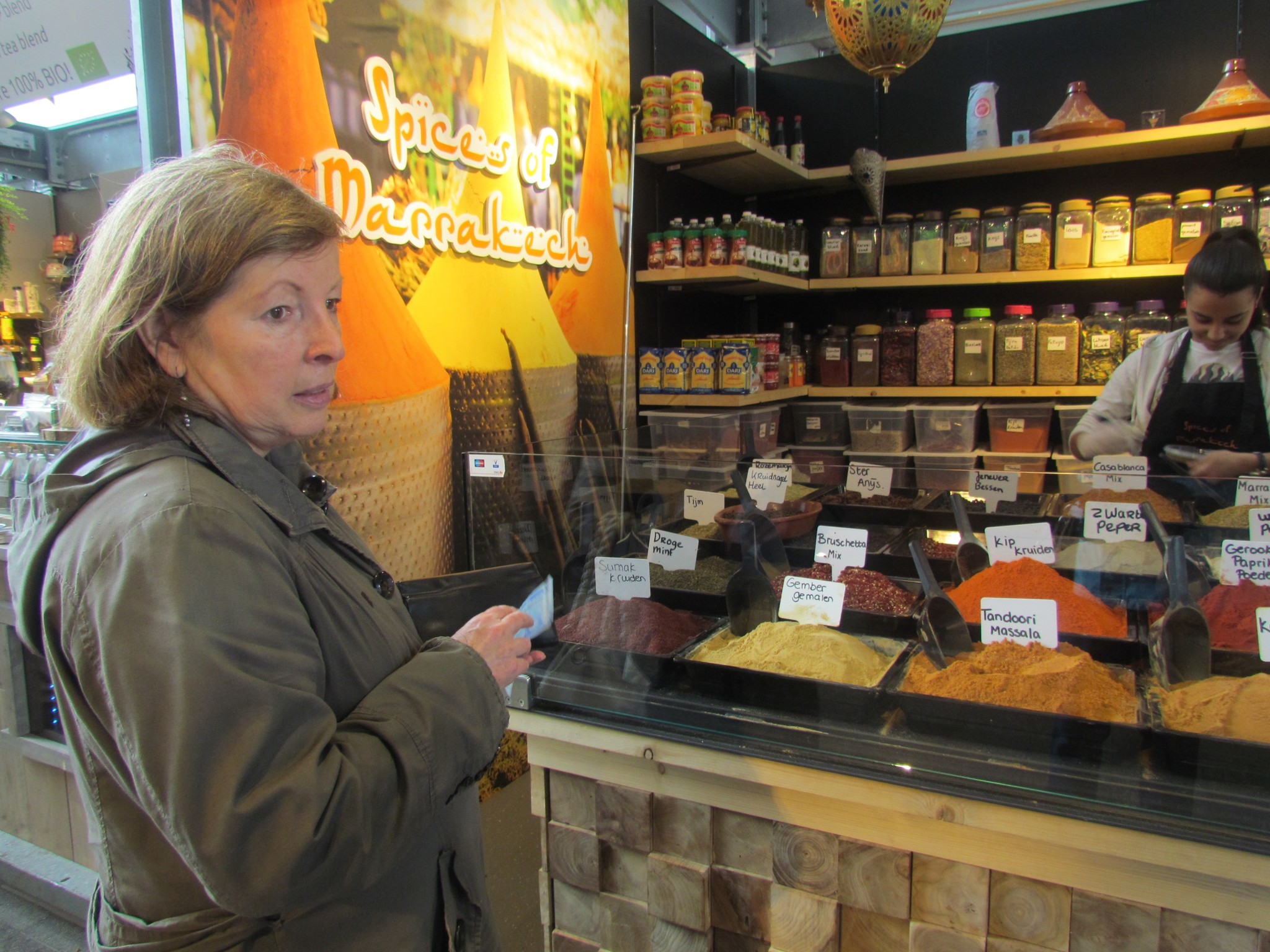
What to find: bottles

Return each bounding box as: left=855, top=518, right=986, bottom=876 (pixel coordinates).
left=644, top=100, right=1269, bottom=395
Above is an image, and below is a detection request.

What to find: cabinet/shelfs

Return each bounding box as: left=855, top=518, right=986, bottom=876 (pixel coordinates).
left=630, top=110, right=1269, bottom=513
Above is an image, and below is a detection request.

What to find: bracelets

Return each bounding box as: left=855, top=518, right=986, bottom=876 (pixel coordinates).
left=1248, top=452, right=1269, bottom=476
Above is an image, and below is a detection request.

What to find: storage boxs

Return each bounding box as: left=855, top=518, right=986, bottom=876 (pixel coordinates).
left=639, top=395, right=1092, bottom=494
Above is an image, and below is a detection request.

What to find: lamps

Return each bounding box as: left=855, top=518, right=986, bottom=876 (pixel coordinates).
left=821, top=1, right=952, bottom=92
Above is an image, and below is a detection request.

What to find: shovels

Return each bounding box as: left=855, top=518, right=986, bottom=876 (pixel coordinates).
left=1144, top=535, right=1215, bottom=686
left=1132, top=501, right=1214, bottom=602
left=906, top=539, right=977, bottom=674
left=728, top=470, right=788, bottom=521
left=947, top=492, right=990, bottom=587
left=725, top=518, right=781, bottom=637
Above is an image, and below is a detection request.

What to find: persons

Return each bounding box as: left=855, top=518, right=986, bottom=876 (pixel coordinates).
left=0, top=137, right=547, bottom=951
left=1067, top=225, right=1270, bottom=510
